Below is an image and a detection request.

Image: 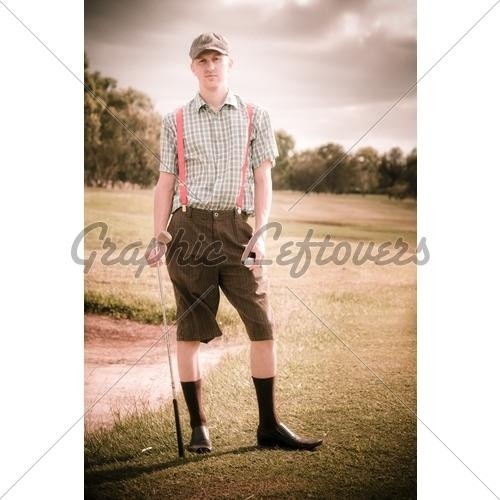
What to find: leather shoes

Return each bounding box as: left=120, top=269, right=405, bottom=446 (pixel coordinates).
left=257, top=423, right=323, bottom=449
left=189, top=424, right=212, bottom=452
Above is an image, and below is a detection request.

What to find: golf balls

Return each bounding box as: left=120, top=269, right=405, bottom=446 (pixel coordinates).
left=244, top=257, right=254, bottom=267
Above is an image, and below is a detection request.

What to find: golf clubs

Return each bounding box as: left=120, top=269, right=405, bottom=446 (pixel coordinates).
left=156, top=230, right=186, bottom=459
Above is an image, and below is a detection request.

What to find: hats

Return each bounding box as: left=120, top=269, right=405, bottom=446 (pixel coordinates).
left=189, top=31, right=231, bottom=60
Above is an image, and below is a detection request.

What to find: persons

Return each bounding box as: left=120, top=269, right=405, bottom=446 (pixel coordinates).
left=145, top=31, right=325, bottom=453
